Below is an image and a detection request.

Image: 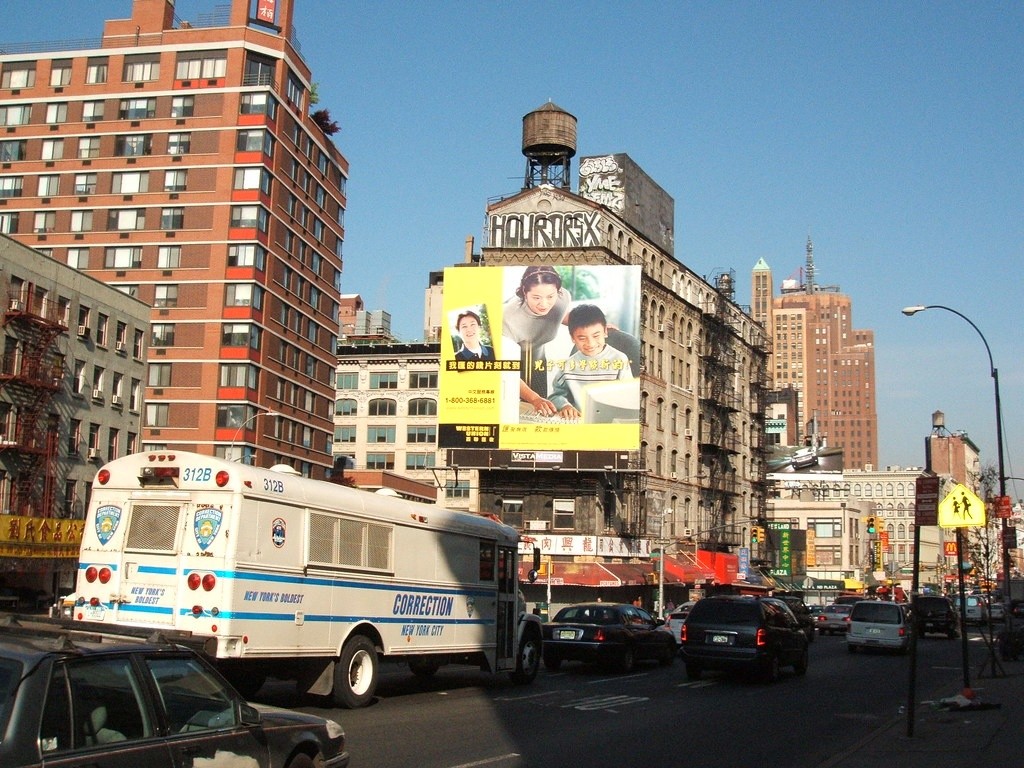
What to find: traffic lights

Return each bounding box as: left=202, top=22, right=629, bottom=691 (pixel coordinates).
left=749, top=526, right=758, bottom=544
left=878, top=518, right=884, bottom=534
left=759, top=529, right=764, bottom=542
left=867, top=516, right=876, bottom=533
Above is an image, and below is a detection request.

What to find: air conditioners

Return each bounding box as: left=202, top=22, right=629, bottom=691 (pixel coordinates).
left=10, top=300, right=24, bottom=311
left=112, top=395, right=121, bottom=405
left=684, top=528, right=692, bottom=537
left=685, top=429, right=693, bottom=437
left=89, top=448, right=100, bottom=458
left=658, top=324, right=665, bottom=333
left=671, top=471, right=677, bottom=479
left=92, top=389, right=103, bottom=399
left=687, top=340, right=693, bottom=348
left=78, top=325, right=90, bottom=338
left=116, top=341, right=126, bottom=352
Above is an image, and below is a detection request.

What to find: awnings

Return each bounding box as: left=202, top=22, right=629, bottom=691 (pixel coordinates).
left=801, top=579, right=845, bottom=591
left=518, top=561, right=681, bottom=586
left=663, top=556, right=714, bottom=584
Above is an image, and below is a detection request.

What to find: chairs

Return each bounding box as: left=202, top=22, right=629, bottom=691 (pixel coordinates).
left=570, top=327, right=640, bottom=380
left=73, top=683, right=128, bottom=748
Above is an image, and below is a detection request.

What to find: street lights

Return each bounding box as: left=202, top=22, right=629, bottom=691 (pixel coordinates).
left=227, top=412, right=281, bottom=460
left=901, top=304, right=1012, bottom=637
left=658, top=466, right=738, bottom=622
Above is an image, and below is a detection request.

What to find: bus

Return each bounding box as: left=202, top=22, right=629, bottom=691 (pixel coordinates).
left=70, top=449, right=545, bottom=706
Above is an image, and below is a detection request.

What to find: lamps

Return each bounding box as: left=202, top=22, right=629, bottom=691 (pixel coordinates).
left=405, top=342, right=412, bottom=348
left=369, top=342, right=375, bottom=348
left=424, top=341, right=430, bottom=347
left=351, top=342, right=358, bottom=349
left=386, top=342, right=394, bottom=348
left=500, top=464, right=509, bottom=468
left=450, top=463, right=460, bottom=469
left=552, top=466, right=561, bottom=469
left=604, top=465, right=614, bottom=470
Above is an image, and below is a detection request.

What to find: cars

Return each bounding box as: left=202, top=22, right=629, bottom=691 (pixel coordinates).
left=771, top=594, right=815, bottom=644
left=818, top=602, right=854, bottom=636
left=665, top=608, right=692, bottom=645
left=677, top=594, right=810, bottom=680
left=844, top=601, right=911, bottom=653
left=1013, top=602, right=1024, bottom=618
left=912, top=595, right=960, bottom=641
left=808, top=604, right=822, bottom=626
left=1, top=609, right=353, bottom=768
left=953, top=593, right=1008, bottom=627
left=544, top=601, right=677, bottom=678
left=834, top=596, right=866, bottom=605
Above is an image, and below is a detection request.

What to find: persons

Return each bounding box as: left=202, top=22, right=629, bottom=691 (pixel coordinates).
left=633, top=595, right=643, bottom=608
left=501, top=266, right=619, bottom=416
left=597, top=597, right=602, bottom=603
left=547, top=303, right=635, bottom=421
left=666, top=598, right=675, bottom=611
left=454, top=310, right=496, bottom=363
